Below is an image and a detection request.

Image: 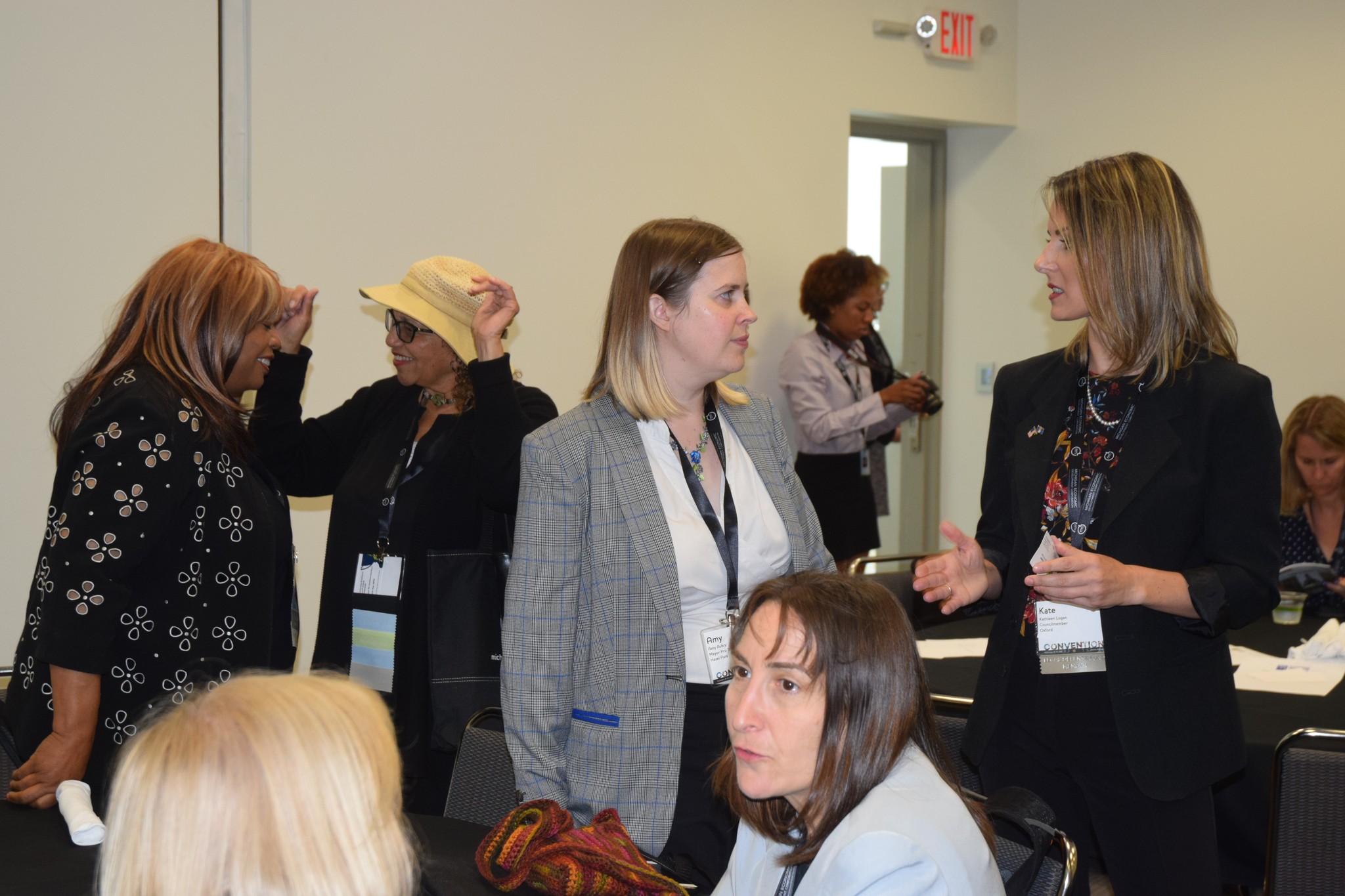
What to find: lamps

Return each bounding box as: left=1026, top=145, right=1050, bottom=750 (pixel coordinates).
left=917, top=9, right=996, bottom=61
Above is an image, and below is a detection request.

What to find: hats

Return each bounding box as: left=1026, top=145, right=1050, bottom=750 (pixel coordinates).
left=359, top=255, right=518, bottom=367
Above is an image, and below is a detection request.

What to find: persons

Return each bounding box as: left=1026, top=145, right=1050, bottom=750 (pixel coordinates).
left=94, top=671, right=423, bottom=896
left=248, top=255, right=559, bottom=818
left=1279, top=396, right=1345, bottom=596
left=705, top=570, right=1008, bottom=896
left=0, top=238, right=302, bottom=807
left=500, top=218, right=837, bottom=896
left=914, top=150, right=1282, bottom=896
left=779, top=249, right=928, bottom=574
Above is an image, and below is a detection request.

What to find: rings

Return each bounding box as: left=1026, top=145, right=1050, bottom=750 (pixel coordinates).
left=942, top=584, right=952, bottom=600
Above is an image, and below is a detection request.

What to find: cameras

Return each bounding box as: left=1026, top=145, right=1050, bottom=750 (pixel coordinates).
left=914, top=375, right=943, bottom=415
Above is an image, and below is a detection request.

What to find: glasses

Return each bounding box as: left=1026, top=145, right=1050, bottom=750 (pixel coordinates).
left=385, top=308, right=434, bottom=343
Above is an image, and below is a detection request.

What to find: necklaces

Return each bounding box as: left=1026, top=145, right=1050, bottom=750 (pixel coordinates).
left=1087, top=362, right=1121, bottom=426
left=423, top=389, right=456, bottom=408
left=669, top=415, right=709, bottom=481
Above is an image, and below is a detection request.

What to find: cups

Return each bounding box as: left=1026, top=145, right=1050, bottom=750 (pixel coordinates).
left=1274, top=591, right=1306, bottom=624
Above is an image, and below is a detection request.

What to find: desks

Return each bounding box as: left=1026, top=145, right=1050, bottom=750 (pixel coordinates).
left=909, top=616, right=1345, bottom=783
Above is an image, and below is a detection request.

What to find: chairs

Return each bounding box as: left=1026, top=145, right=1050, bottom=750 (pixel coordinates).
left=404, top=546, right=1345, bottom=895
